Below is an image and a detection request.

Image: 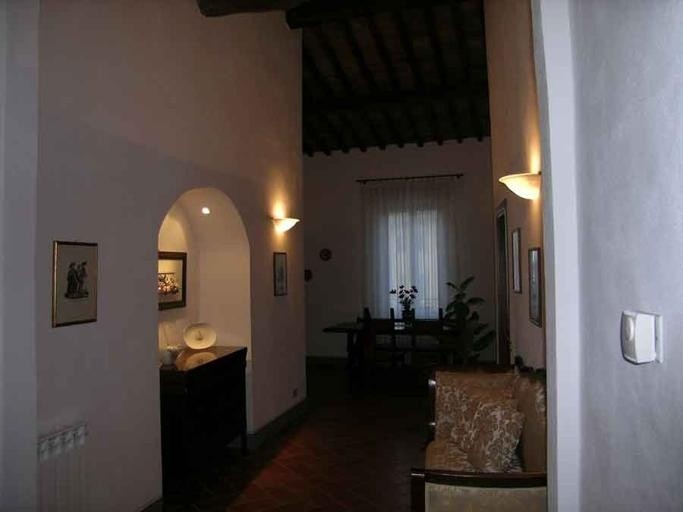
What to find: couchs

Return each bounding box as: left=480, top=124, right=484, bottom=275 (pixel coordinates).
left=410, top=356, right=548, bottom=512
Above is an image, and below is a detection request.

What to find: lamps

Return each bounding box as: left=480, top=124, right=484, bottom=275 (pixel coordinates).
left=499, top=171, right=542, bottom=201
left=268, top=216, right=300, bottom=234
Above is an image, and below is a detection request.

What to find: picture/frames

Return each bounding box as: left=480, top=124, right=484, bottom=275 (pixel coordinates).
left=512, top=227, right=521, bottom=293
left=273, top=252, right=287, bottom=296
left=158, top=252, right=186, bottom=311
left=52, top=241, right=98, bottom=328
left=528, top=247, right=542, bottom=328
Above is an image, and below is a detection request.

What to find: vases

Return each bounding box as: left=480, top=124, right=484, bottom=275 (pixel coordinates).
left=402, top=308, right=415, bottom=325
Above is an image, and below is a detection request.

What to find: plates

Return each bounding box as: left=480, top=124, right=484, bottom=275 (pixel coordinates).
left=183, top=322, right=217, bottom=350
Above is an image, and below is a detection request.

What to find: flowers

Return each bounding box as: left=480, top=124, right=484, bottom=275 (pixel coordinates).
left=389, top=285, right=418, bottom=311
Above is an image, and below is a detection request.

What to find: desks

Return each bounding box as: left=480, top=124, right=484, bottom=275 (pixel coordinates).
left=161, top=345, right=247, bottom=505
left=323, top=309, right=479, bottom=393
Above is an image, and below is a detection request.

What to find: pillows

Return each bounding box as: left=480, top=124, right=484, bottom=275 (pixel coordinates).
left=447, top=383, right=526, bottom=472
